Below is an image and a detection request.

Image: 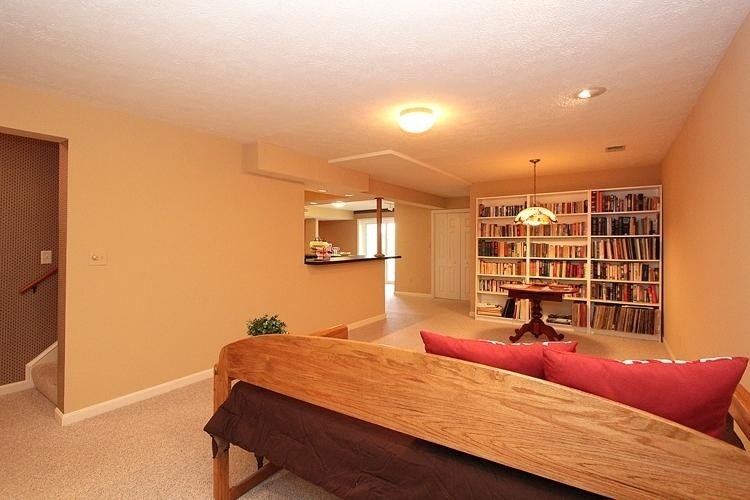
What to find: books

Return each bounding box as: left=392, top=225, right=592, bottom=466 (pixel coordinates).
left=477, top=191, right=660, bottom=335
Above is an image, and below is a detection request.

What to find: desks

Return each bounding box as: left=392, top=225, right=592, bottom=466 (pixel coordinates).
left=500, top=284, right=579, bottom=342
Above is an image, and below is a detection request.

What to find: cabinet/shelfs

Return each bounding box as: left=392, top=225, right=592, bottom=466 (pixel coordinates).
left=475, top=194, right=528, bottom=327
left=528, top=189, right=589, bottom=336
left=590, top=185, right=664, bottom=344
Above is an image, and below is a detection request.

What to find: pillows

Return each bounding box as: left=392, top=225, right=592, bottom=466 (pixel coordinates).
left=540, top=347, right=749, bottom=450
left=419, top=330, right=579, bottom=377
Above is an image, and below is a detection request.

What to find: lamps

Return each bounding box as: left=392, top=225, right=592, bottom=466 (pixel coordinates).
left=514, top=158, right=559, bottom=227
left=397, top=107, right=433, bottom=135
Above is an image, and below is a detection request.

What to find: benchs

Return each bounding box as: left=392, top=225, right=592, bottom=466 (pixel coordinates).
left=203, top=324, right=749, bottom=500
